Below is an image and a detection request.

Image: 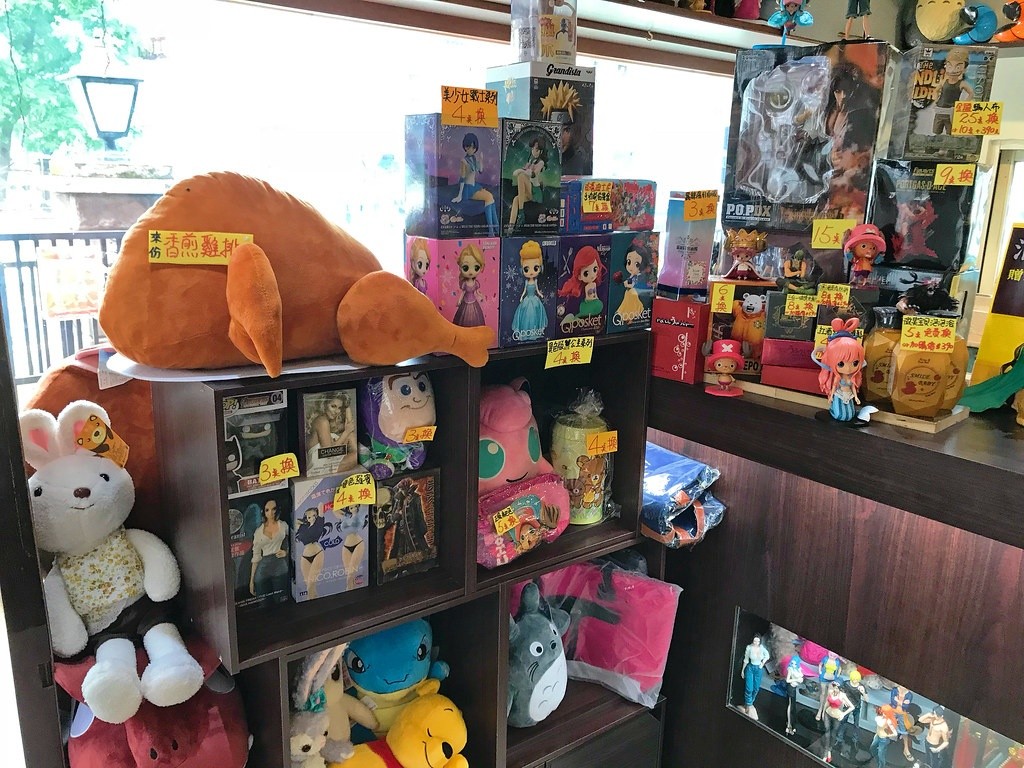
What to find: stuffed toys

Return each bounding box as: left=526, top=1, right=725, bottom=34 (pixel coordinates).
left=20, top=343, right=254, bottom=768
left=506, top=581, right=573, bottom=729
left=360, top=370, right=436, bottom=480
left=288, top=619, right=471, bottom=768
left=98, top=171, right=496, bottom=379
left=477, top=374, right=570, bottom=569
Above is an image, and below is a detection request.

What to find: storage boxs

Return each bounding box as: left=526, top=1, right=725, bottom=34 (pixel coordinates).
left=221, top=388, right=441, bottom=614
left=704, top=275, right=777, bottom=375
left=761, top=339, right=827, bottom=395
left=404, top=61, right=660, bottom=356
left=759, top=651, right=940, bottom=754
left=716, top=39, right=999, bottom=284
left=849, top=264, right=980, bottom=347
left=765, top=289, right=880, bottom=341
left=651, top=297, right=711, bottom=385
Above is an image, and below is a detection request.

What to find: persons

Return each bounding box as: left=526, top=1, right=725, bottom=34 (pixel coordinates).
left=811, top=318, right=867, bottom=422
left=687, top=0, right=814, bottom=35
left=844, top=0, right=875, bottom=40
left=305, top=390, right=358, bottom=477
left=784, top=650, right=949, bottom=768
left=843, top=224, right=887, bottom=285
left=721, top=228, right=773, bottom=281
left=776, top=249, right=816, bottom=294
left=740, top=633, right=770, bottom=714
left=707, top=339, right=745, bottom=390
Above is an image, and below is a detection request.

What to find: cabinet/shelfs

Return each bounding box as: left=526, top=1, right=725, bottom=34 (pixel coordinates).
left=151, top=328, right=652, bottom=677
left=236, top=537, right=667, bottom=768
